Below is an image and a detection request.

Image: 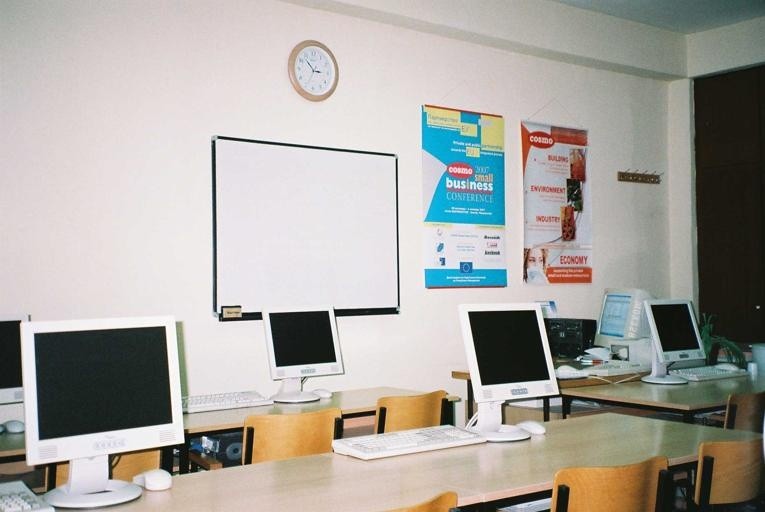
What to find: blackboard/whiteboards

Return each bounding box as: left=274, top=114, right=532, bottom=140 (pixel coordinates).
left=211, top=135, right=401, bottom=321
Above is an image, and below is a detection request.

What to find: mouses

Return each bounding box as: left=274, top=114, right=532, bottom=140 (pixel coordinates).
left=133, top=470, right=173, bottom=491
left=717, top=363, right=739, bottom=371
left=558, top=365, right=579, bottom=374
left=312, top=389, right=331, bottom=398
left=5, top=421, right=25, bottom=433
left=515, top=422, right=547, bottom=435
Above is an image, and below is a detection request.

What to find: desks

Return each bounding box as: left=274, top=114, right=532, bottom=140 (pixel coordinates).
left=450, top=357, right=764, bottom=427
left=6, top=378, right=463, bottom=462
left=39, top=410, right=763, bottom=512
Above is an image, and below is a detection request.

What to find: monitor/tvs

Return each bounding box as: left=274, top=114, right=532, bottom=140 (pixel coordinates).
left=640, top=299, right=707, bottom=384
left=594, top=288, right=657, bottom=361
left=19, top=315, right=185, bottom=509
left=458, top=303, right=559, bottom=443
left=0, top=314, right=31, bottom=434
left=262, top=306, right=344, bottom=403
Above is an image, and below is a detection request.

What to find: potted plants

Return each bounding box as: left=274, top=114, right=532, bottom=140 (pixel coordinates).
left=699, top=312, right=748, bottom=370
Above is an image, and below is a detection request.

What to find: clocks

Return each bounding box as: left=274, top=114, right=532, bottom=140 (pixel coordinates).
left=286, top=40, right=339, bottom=103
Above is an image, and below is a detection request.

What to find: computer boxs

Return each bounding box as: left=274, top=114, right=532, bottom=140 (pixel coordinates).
left=191, top=432, right=243, bottom=468
left=544, top=318, right=596, bottom=358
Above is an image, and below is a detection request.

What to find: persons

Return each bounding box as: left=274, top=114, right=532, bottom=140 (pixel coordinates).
left=523, top=248, right=547, bottom=283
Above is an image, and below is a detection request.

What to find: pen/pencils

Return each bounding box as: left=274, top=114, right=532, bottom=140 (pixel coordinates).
left=580, top=358, right=603, bottom=364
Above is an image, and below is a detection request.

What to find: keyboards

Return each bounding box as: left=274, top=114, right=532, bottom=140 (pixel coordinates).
left=584, top=361, right=650, bottom=376
left=669, top=366, right=734, bottom=382
left=182, top=391, right=274, bottom=414
left=0, top=480, right=55, bottom=512
left=331, top=424, right=487, bottom=460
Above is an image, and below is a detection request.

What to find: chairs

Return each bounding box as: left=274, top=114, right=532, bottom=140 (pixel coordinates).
left=383, top=489, right=462, bottom=512
left=548, top=454, right=677, bottom=512
left=188, top=405, right=345, bottom=474
left=706, top=391, right=763, bottom=434
left=43, top=447, right=163, bottom=495
left=674, top=436, right=764, bottom=512
left=373, top=388, right=453, bottom=434
